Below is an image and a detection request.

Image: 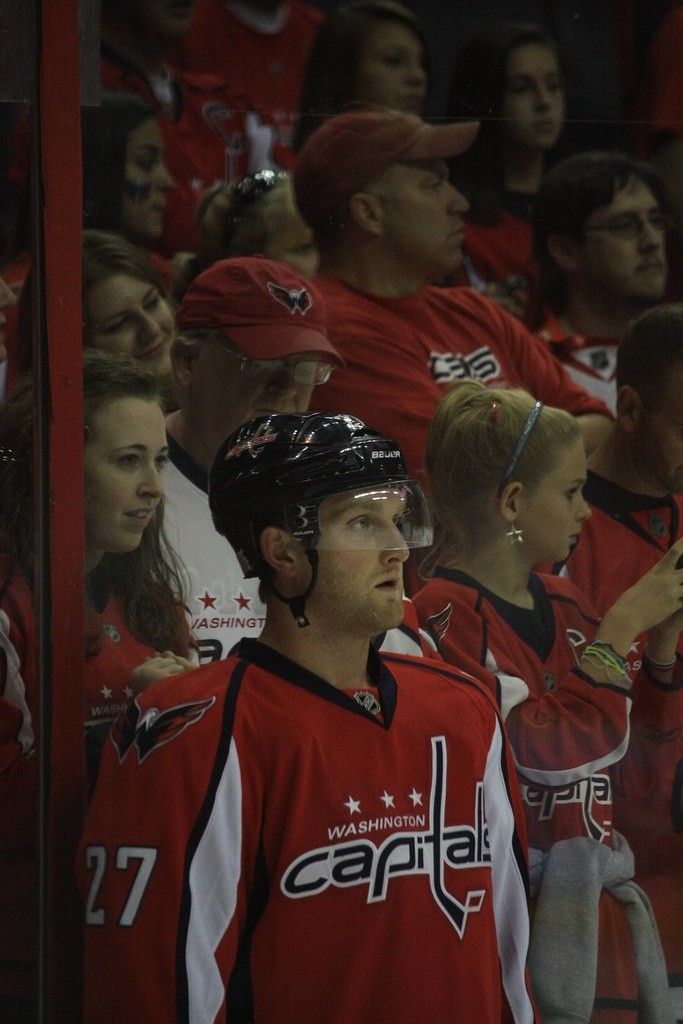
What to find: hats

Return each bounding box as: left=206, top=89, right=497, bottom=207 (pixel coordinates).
left=176, top=252, right=348, bottom=371
left=291, top=106, right=480, bottom=231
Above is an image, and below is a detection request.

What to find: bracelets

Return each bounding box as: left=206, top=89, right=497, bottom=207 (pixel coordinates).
left=580, top=638, right=625, bottom=684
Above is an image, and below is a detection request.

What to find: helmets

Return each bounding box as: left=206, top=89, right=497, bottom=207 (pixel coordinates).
left=206, top=410, right=432, bottom=577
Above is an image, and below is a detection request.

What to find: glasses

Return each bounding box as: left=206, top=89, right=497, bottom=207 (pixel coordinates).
left=581, top=216, right=673, bottom=236
left=197, top=331, right=336, bottom=385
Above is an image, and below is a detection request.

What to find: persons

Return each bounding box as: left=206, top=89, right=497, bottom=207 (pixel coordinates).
left=450, top=23, right=615, bottom=319
left=532, top=151, right=669, bottom=423
left=82, top=85, right=171, bottom=247
left=409, top=380, right=683, bottom=1024
left=1, top=344, right=200, bottom=1022
left=291, top=1, right=435, bottom=156
left=9, top=3, right=292, bottom=264
left=533, top=298, right=682, bottom=685
left=186, top=1, right=324, bottom=178
left=156, top=255, right=346, bottom=498
left=86, top=406, right=540, bottom=1024
left=296, top=103, right=614, bottom=522
left=16, top=227, right=181, bottom=415
